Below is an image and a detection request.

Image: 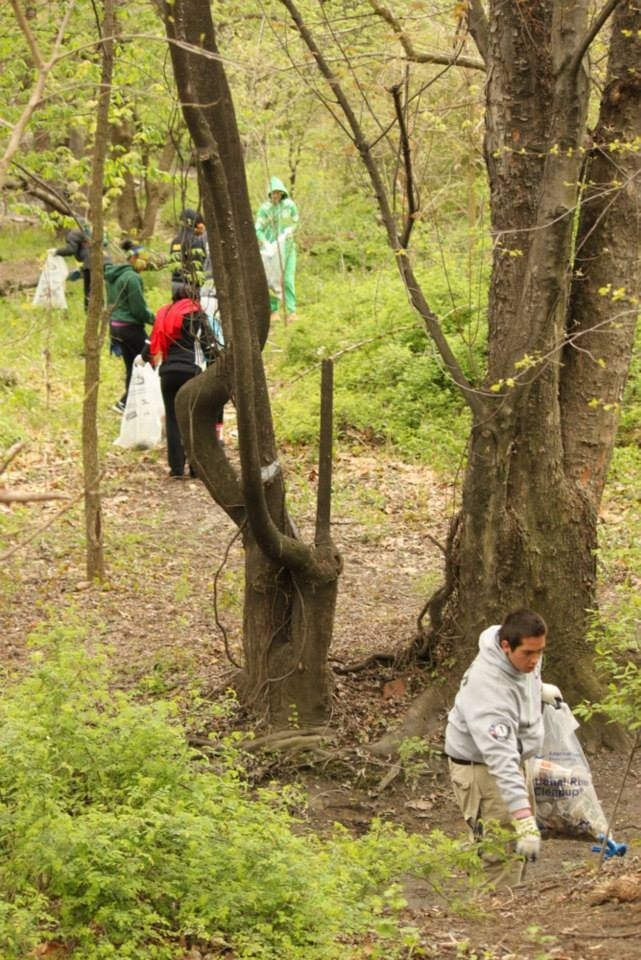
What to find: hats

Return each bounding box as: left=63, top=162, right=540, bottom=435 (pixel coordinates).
left=125, top=246, right=149, bottom=260
left=179, top=208, right=196, bottom=225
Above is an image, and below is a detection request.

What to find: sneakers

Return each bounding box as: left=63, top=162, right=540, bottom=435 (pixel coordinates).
left=191, top=468, right=198, bottom=478
left=169, top=467, right=183, bottom=480
left=112, top=401, right=126, bottom=414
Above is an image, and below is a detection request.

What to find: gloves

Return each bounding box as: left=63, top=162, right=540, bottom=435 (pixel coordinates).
left=49, top=248, right=56, bottom=258
left=137, top=357, right=148, bottom=367
left=514, top=815, right=540, bottom=861
left=540, top=681, right=563, bottom=710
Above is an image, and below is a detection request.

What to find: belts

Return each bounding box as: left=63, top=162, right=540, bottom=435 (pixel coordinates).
left=449, top=757, right=483, bottom=766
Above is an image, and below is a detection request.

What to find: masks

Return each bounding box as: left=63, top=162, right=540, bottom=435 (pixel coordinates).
left=134, top=258, right=146, bottom=272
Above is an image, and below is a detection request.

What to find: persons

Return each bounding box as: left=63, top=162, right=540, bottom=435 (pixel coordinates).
left=445, top=609, right=563, bottom=864
left=56, top=208, right=226, bottom=478
left=255, top=176, right=300, bottom=321
